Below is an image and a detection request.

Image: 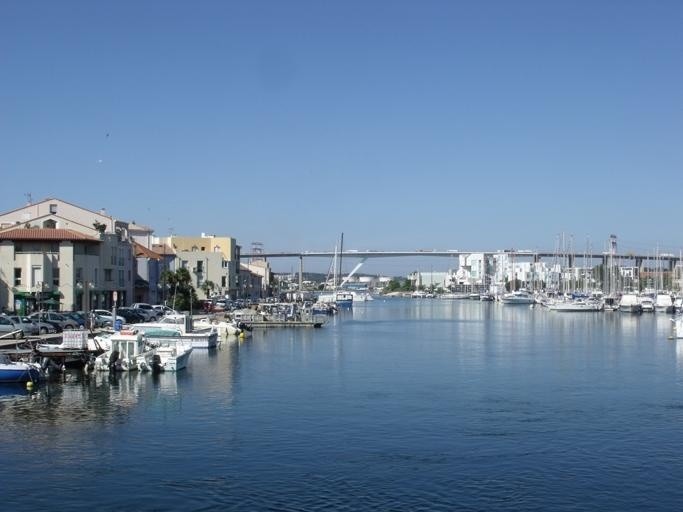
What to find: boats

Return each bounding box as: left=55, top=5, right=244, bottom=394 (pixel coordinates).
left=93, top=329, right=153, bottom=372
left=128, top=314, right=218, bottom=349
left=5, top=328, right=107, bottom=368
left=232, top=244, right=374, bottom=327
left=0, top=354, right=48, bottom=384
left=135, top=329, right=193, bottom=372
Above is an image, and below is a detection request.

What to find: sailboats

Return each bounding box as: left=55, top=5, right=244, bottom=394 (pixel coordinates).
left=383, top=233, right=682, bottom=339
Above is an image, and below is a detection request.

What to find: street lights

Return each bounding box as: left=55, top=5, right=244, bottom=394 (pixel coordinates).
left=156, top=282, right=171, bottom=303
left=75, top=279, right=95, bottom=328
left=35, top=280, right=49, bottom=310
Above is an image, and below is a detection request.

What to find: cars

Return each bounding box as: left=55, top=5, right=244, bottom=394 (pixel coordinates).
left=0, top=302, right=176, bottom=340
left=201, top=297, right=276, bottom=310
